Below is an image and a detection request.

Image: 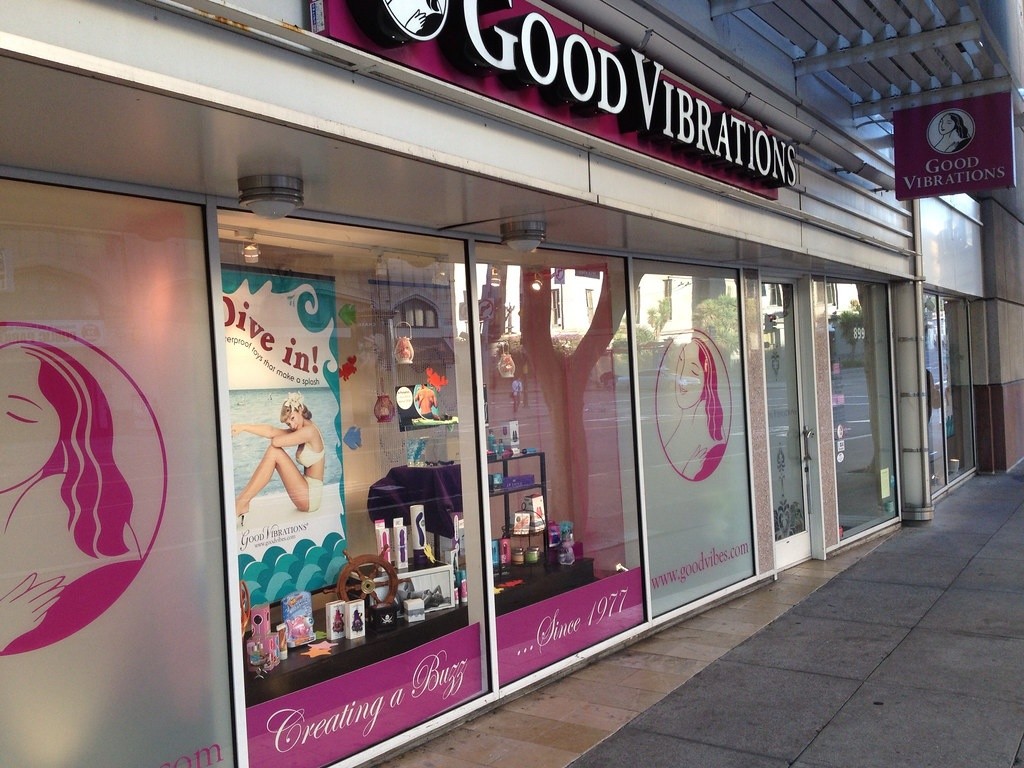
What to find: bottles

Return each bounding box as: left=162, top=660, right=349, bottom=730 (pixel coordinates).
left=519, top=447, right=537, bottom=455
left=488, top=429, right=505, bottom=455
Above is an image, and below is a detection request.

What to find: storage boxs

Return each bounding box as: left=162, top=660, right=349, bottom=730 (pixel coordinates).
left=514, top=509, right=530, bottom=533
left=345, top=599, right=365, bottom=640
left=524, top=492, right=546, bottom=532
left=345, top=559, right=454, bottom=615
left=500, top=419, right=520, bottom=454
left=326, top=599, right=346, bottom=639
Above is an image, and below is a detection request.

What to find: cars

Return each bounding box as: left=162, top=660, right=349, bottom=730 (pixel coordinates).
left=617, top=367, right=701, bottom=391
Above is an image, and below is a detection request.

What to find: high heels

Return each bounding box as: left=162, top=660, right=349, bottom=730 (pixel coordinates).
left=236, top=511, right=248, bottom=526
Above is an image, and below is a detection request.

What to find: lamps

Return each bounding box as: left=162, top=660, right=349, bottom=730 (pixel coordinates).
left=242, top=237, right=260, bottom=263
left=237, top=174, right=304, bottom=221
left=491, top=268, right=501, bottom=286
left=499, top=220, right=546, bottom=255
left=532, top=272, right=543, bottom=290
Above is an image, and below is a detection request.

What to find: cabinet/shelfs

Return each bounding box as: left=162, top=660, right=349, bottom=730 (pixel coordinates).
left=367, top=450, right=550, bottom=567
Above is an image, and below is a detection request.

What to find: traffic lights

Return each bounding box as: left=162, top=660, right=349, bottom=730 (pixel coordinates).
left=768, top=315, right=777, bottom=332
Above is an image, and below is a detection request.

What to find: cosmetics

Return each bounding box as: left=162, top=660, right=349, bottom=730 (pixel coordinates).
left=492, top=537, right=541, bottom=567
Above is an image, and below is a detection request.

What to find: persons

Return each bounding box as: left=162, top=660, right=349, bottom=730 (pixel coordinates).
left=233, top=390, right=326, bottom=526
left=415, top=384, right=440, bottom=420
